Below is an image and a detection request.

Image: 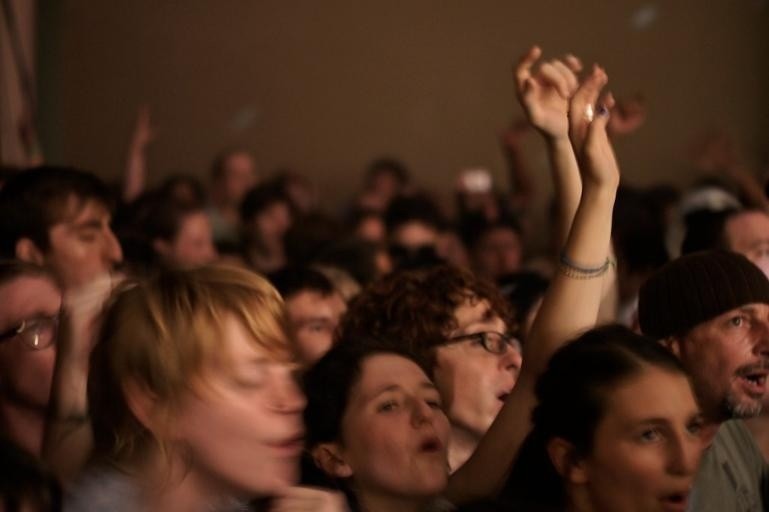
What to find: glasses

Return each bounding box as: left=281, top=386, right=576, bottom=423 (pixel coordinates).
left=2, top=318, right=58, bottom=350
left=434, top=330, right=523, bottom=354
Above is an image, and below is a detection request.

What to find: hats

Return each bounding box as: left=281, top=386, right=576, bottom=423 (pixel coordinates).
left=638, top=251, right=769, bottom=338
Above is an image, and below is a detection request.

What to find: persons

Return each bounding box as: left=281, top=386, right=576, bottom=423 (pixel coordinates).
left=1, top=46, right=769, bottom=510
